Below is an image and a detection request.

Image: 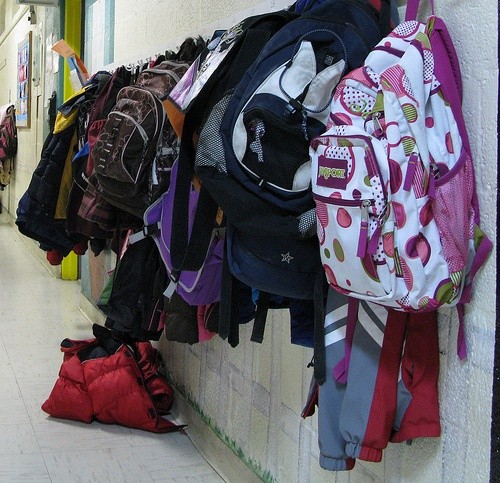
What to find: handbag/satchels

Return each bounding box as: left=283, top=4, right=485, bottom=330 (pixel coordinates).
left=140, top=155, right=228, bottom=306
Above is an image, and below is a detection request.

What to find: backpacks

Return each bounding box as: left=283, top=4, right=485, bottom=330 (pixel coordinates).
left=95, top=44, right=193, bottom=219
left=218, top=0, right=393, bottom=219
left=0, top=105, right=18, bottom=162
left=309, top=0, right=483, bottom=319
